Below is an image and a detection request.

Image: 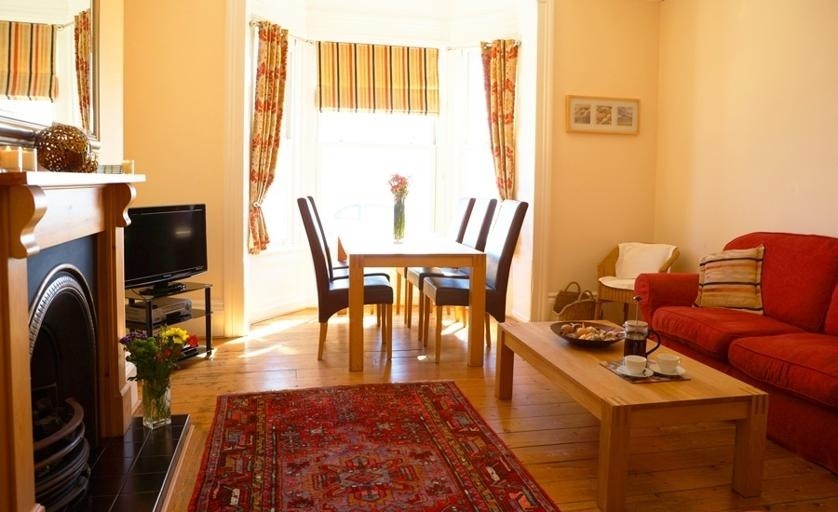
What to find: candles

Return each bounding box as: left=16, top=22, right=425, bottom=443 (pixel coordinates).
left=22, top=148, right=38, bottom=172
left=123, top=160, right=133, bottom=173
left=0, top=145, right=22, bottom=171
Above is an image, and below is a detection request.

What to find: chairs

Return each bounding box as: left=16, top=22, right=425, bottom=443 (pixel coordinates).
left=423, top=198, right=529, bottom=366
left=298, top=196, right=395, bottom=369
left=595, top=242, right=680, bottom=320
left=307, top=196, right=391, bottom=329
left=393, top=194, right=474, bottom=324
left=406, top=198, right=498, bottom=343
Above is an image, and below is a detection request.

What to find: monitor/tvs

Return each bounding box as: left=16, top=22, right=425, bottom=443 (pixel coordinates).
left=125, top=203, right=209, bottom=295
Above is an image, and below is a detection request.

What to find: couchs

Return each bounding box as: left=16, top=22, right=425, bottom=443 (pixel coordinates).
left=635, top=232, right=838, bottom=477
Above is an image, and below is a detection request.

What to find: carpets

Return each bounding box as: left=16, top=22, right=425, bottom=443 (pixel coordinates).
left=188, top=380, right=561, bottom=512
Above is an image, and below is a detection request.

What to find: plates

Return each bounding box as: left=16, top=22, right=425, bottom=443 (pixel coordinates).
left=550, top=322, right=625, bottom=347
left=617, top=364, right=685, bottom=378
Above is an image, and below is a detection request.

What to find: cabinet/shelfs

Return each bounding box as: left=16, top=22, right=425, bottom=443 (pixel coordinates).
left=123, top=281, right=215, bottom=367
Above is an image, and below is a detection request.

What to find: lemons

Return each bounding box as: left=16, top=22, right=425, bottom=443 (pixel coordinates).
left=561, top=324, right=597, bottom=339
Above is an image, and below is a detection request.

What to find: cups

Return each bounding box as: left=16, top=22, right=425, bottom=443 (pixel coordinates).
left=625, top=355, right=681, bottom=373
left=622, top=320, right=661, bottom=358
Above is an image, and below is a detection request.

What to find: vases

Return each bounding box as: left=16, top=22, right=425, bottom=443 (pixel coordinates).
left=141, top=379, right=173, bottom=430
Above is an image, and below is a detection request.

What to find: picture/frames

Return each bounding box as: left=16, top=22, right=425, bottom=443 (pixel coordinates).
left=565, top=95, right=640, bottom=136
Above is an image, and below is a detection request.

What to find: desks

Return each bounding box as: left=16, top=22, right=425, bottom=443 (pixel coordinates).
left=346, top=235, right=486, bottom=370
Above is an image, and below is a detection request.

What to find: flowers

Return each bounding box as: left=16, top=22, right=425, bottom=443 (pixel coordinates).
left=388, top=174, right=409, bottom=239
left=120, top=327, right=200, bottom=419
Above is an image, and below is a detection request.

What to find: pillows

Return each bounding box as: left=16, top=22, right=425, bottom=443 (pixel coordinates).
left=692, top=243, right=765, bottom=316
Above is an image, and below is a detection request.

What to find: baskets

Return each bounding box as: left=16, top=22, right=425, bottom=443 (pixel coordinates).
left=551, top=280, right=596, bottom=321
